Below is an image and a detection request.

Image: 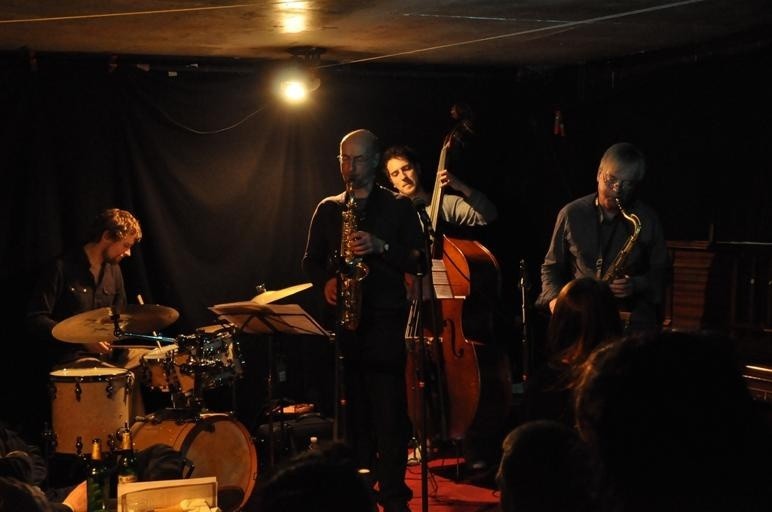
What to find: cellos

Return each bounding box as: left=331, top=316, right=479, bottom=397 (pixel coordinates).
left=407, top=105, right=512, bottom=447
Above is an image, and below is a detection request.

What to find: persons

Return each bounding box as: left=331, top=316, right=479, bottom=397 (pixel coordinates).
left=260, top=462, right=371, bottom=512
left=379, top=144, right=500, bottom=477
left=493, top=419, right=616, bottom=512
left=517, top=276, right=624, bottom=421
left=572, top=327, right=772, bottom=511
left=298, top=126, right=434, bottom=512
left=0, top=421, right=73, bottom=512
left=534, top=142, right=670, bottom=335
left=23, top=208, right=144, bottom=482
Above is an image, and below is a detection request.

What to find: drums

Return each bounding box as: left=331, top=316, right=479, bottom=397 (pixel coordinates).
left=143, top=334, right=245, bottom=396
left=128, top=408, right=257, bottom=512
left=49, top=368, right=132, bottom=454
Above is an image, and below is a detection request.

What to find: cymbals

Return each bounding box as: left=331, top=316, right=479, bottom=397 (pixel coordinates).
left=218, top=283, right=313, bottom=321
left=51, top=304, right=179, bottom=343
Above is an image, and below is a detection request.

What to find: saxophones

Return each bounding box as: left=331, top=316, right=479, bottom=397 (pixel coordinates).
left=601, top=198, right=642, bottom=288
left=332, top=181, right=369, bottom=331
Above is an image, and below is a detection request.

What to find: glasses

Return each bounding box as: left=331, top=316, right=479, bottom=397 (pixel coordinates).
left=602, top=169, right=636, bottom=192
left=338, top=153, right=375, bottom=165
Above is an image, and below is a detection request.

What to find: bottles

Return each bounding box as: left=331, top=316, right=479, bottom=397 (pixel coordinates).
left=116, top=429, right=140, bottom=486
left=85, top=440, right=111, bottom=512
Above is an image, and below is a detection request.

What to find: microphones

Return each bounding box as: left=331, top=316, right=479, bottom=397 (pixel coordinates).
left=519, top=261, right=531, bottom=291
left=413, top=197, right=435, bottom=237
left=111, top=287, right=123, bottom=314
